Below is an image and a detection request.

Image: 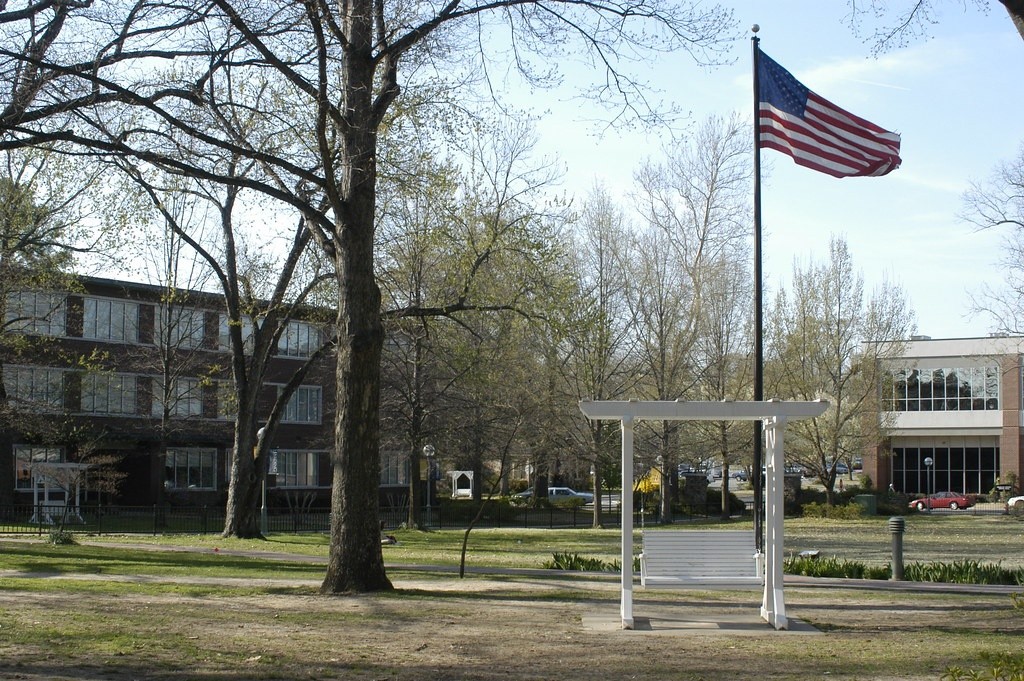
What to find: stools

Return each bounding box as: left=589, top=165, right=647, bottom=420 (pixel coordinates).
left=798, top=550, right=819, bottom=560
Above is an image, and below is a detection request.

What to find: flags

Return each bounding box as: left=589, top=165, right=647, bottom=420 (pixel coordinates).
left=755, top=47, right=902, bottom=180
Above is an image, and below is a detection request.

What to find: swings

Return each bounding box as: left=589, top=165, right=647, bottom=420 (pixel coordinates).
left=635, top=420, right=765, bottom=590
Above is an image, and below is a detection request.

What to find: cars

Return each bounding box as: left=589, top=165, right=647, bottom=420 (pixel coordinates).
left=511, top=487, right=594, bottom=505
left=733, top=458, right=863, bottom=481
left=712, top=468, right=722, bottom=478
left=908, top=491, right=976, bottom=512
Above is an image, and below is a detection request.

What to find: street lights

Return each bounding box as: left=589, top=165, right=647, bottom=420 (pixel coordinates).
left=924, top=457, right=933, bottom=514
left=257, top=428, right=269, bottom=536
left=656, top=455, right=664, bottom=506
left=525, top=464, right=533, bottom=488
left=423, top=444, right=435, bottom=526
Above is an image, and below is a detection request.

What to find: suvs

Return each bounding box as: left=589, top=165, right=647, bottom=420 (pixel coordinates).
left=677, top=464, right=692, bottom=477
